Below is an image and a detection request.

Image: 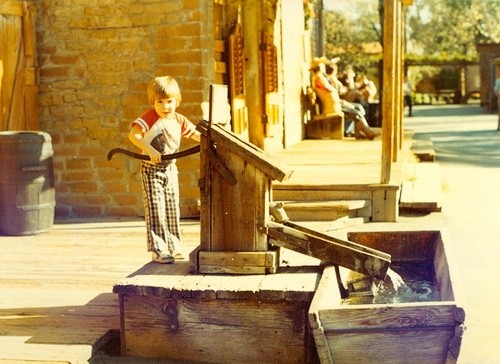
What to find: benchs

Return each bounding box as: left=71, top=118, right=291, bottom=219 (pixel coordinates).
left=306, top=85, right=344, bottom=140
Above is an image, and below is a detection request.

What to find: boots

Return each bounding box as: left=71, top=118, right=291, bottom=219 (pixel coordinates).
left=355, top=118, right=382, bottom=141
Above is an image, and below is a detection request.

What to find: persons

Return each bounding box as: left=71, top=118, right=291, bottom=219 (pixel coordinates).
left=493, top=78, right=500, bottom=130
left=403, top=74, right=416, bottom=117
left=308, top=55, right=381, bottom=141
left=128, top=76, right=202, bottom=264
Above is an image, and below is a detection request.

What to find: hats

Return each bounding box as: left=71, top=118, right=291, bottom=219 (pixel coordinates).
left=308, top=56, right=327, bottom=70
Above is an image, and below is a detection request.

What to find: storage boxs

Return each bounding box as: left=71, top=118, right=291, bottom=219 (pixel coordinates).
left=0, top=130, right=56, bottom=237
left=308, top=221, right=466, bottom=364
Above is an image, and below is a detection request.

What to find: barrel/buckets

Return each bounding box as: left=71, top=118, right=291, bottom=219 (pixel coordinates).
left=0, top=131, right=56, bottom=235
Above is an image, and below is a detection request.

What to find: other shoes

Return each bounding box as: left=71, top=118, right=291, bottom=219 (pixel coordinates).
left=151, top=249, right=185, bottom=263
